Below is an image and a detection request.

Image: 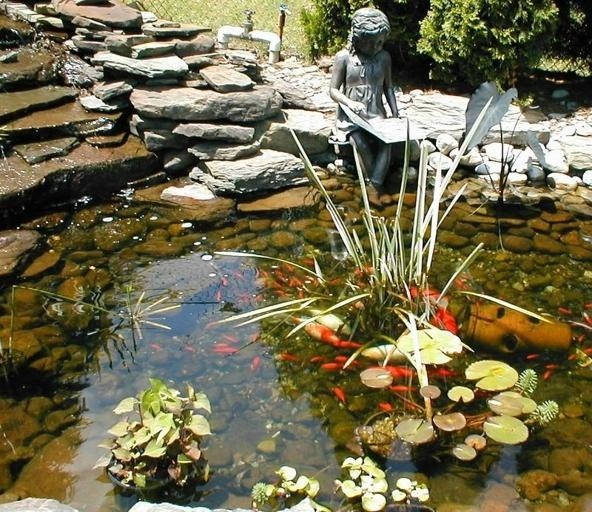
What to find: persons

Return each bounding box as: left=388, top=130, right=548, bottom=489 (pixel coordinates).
left=329, top=8, right=398, bottom=207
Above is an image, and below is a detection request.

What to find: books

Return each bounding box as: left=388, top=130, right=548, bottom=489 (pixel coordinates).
left=339, top=102, right=426, bottom=144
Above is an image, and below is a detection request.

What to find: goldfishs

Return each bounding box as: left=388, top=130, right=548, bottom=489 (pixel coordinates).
left=147, top=256, right=492, bottom=414
